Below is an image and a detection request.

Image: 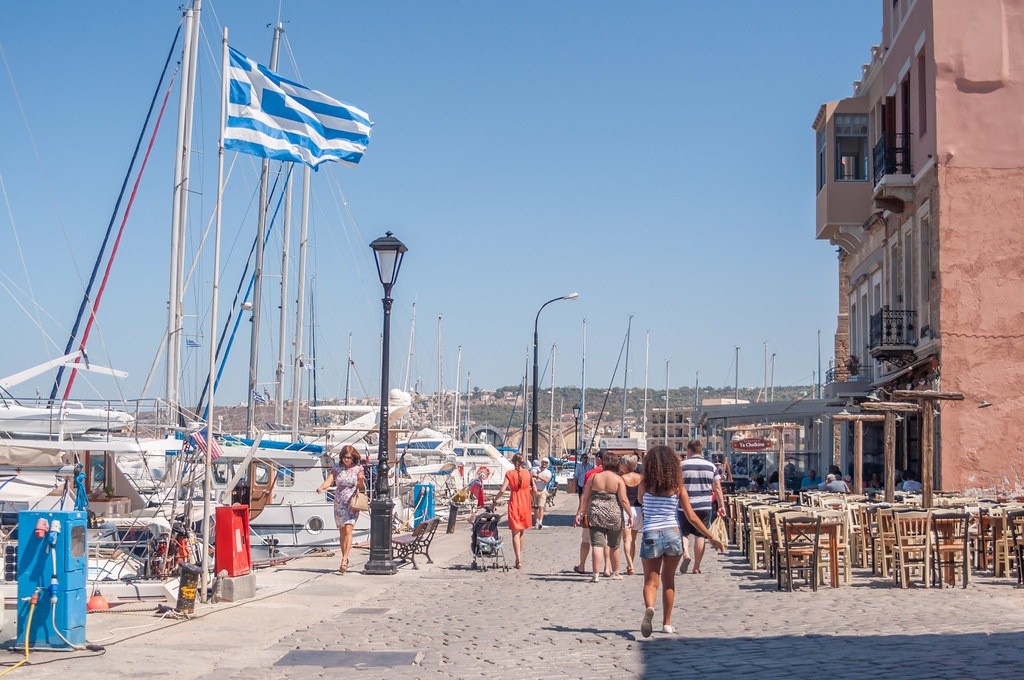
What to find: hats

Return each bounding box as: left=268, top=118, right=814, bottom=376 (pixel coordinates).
left=595, top=451, right=604, bottom=457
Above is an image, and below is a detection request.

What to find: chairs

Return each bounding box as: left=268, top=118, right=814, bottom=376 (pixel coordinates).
left=721, top=489, right=1024, bottom=592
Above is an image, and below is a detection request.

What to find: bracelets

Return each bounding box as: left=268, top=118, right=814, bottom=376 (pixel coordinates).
left=537, top=475, right=538, bottom=478
left=493, top=500, right=496, bottom=503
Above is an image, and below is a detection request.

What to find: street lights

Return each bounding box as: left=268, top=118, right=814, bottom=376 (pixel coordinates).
left=771, top=353, right=780, bottom=403
left=664, top=359, right=671, bottom=446
left=531, top=289, right=588, bottom=464
left=625, top=311, right=634, bottom=440
left=363, top=230, right=413, bottom=575
left=736, top=346, right=746, bottom=405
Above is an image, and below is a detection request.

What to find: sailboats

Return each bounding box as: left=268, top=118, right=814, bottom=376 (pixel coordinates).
left=4, top=0, right=548, bottom=577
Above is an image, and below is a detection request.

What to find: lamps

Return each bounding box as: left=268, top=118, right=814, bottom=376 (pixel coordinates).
left=813, top=387, right=993, bottom=427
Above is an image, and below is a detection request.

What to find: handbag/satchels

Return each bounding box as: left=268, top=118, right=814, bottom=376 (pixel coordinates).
left=350, top=485, right=368, bottom=511
left=707, top=514, right=728, bottom=549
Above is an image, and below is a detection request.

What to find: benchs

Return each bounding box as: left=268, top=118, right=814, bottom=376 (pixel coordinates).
left=391, top=516, right=440, bottom=569
left=545, top=486, right=558, bottom=507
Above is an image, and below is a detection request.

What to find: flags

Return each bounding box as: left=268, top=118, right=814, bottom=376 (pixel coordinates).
left=224, top=45, right=374, bottom=172
left=255, top=392, right=265, bottom=405
left=192, top=426, right=225, bottom=461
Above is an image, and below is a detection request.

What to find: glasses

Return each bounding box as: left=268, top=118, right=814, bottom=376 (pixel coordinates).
left=342, top=456, right=352, bottom=460
left=582, top=457, right=589, bottom=458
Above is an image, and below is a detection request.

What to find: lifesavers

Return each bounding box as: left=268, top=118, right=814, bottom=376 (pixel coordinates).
left=152, top=547, right=179, bottom=576
left=477, top=466, right=490, bottom=479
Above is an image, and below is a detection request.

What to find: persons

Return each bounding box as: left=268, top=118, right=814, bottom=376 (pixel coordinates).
left=532, top=458, right=552, bottom=529
left=863, top=472, right=879, bottom=487
left=491, top=454, right=538, bottom=568
left=316, top=445, right=364, bottom=574
left=895, top=468, right=921, bottom=490
left=676, top=440, right=727, bottom=573
left=749, top=472, right=787, bottom=491
left=573, top=451, right=644, bottom=583
left=801, top=469, right=853, bottom=494
left=638, top=446, right=725, bottom=637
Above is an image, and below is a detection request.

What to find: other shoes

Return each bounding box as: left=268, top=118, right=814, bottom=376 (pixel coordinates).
left=340, top=558, right=349, bottom=573
left=640, top=606, right=655, bottom=637
left=516, top=561, right=521, bottom=568
left=612, top=571, right=624, bottom=579
left=590, top=576, right=599, bottom=583
left=663, top=625, right=674, bottom=633
left=535, top=522, right=542, bottom=530
left=626, top=565, right=634, bottom=575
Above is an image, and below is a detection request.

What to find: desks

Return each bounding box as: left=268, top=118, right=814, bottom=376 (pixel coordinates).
left=974, top=514, right=1022, bottom=577
left=777, top=520, right=844, bottom=588
left=888, top=516, right=963, bottom=586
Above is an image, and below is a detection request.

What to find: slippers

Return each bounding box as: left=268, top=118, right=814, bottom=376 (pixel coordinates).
left=574, top=565, right=585, bottom=574
left=680, top=558, right=691, bottom=573
left=693, top=568, right=701, bottom=574
left=603, top=572, right=611, bottom=577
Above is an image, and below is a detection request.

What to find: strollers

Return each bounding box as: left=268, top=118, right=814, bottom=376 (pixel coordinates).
left=467, top=505, right=512, bottom=574
